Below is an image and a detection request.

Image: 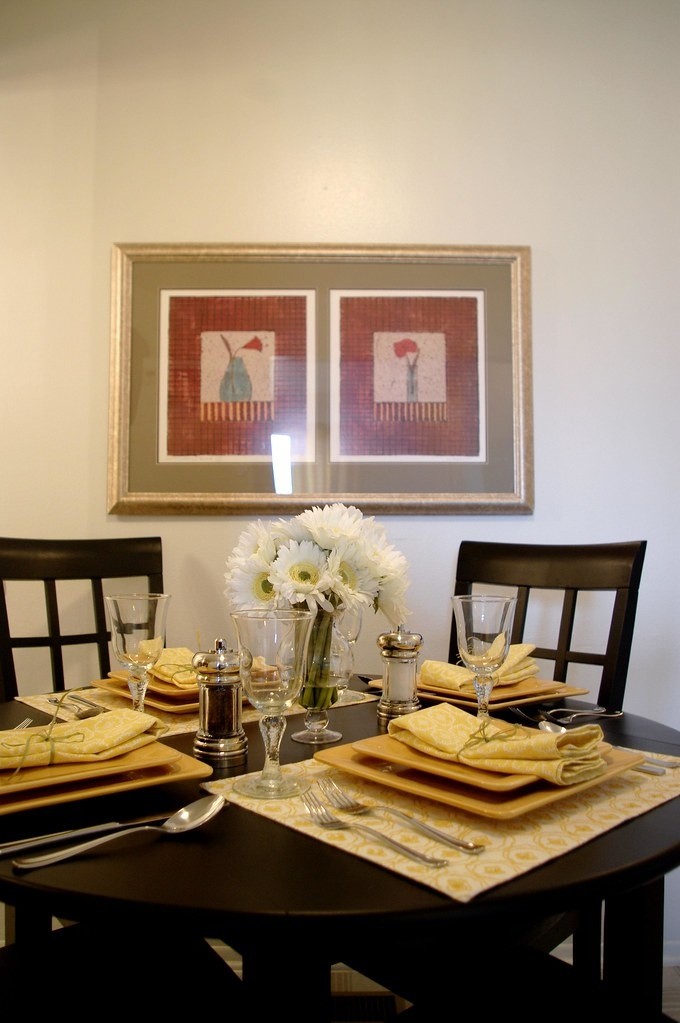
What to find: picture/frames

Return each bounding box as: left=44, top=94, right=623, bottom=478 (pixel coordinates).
left=107, top=241, right=535, bottom=516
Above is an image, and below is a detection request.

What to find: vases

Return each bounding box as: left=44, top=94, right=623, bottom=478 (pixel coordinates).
left=280, top=621, right=354, bottom=743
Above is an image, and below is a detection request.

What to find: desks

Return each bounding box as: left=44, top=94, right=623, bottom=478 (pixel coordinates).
left=0, top=674, right=680, bottom=1023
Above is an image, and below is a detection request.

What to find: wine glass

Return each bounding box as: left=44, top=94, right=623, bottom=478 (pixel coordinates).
left=334, top=606, right=365, bottom=702
left=104, top=592, right=173, bottom=733
left=451, top=594, right=519, bottom=734
left=230, top=610, right=317, bottom=800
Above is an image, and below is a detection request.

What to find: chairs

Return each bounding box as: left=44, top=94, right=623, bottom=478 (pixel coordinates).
left=449, top=540, right=650, bottom=709
left=0, top=536, right=167, bottom=703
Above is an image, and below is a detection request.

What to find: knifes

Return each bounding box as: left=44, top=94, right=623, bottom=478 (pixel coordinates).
left=0, top=801, right=231, bottom=855
left=70, top=694, right=164, bottom=731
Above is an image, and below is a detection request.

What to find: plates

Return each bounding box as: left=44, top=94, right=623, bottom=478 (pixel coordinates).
left=0, top=740, right=214, bottom=815
left=313, top=734, right=645, bottom=819
left=92, top=666, right=282, bottom=715
left=368, top=675, right=590, bottom=710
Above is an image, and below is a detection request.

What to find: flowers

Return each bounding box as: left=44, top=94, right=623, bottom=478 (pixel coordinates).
left=224, top=503, right=413, bottom=709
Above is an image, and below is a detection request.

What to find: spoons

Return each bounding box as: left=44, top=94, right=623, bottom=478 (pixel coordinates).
left=47, top=697, right=103, bottom=719
left=538, top=721, right=666, bottom=776
left=358, top=674, right=374, bottom=685
left=13, top=794, right=226, bottom=871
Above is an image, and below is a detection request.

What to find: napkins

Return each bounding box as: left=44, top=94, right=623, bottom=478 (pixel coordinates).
left=0, top=707, right=171, bottom=770
left=124, top=636, right=279, bottom=688
left=421, top=632, right=541, bottom=693
left=387, top=701, right=614, bottom=787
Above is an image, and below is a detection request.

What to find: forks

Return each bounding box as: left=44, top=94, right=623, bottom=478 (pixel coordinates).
left=14, top=717, right=33, bottom=730
left=317, top=777, right=485, bottom=855
left=509, top=706, right=606, bottom=723
left=538, top=710, right=624, bottom=725
left=301, top=791, right=448, bottom=868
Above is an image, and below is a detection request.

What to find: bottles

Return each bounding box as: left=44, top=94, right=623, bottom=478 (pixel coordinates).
left=191, top=638, right=251, bottom=768
left=375, top=619, right=424, bottom=724
